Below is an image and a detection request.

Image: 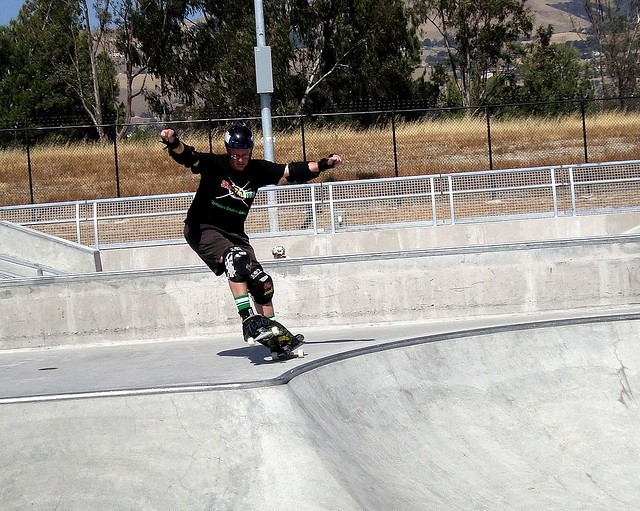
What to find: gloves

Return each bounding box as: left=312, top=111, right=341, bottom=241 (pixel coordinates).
left=158, top=127, right=180, bottom=150
left=317, top=153, right=336, bottom=171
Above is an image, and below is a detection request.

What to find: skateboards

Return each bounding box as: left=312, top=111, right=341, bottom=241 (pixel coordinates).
left=242, top=315, right=305, bottom=361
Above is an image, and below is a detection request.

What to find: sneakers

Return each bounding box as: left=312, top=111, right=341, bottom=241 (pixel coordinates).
left=237, top=307, right=262, bottom=342
left=271, top=334, right=304, bottom=361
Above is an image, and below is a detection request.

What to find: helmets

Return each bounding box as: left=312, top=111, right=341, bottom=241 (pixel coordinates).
left=224, top=126, right=254, bottom=149
left=272, top=245, right=286, bottom=258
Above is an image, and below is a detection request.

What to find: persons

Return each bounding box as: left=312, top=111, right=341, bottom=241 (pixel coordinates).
left=158, top=125, right=344, bottom=342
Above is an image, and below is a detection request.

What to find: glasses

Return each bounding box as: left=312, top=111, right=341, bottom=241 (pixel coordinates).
left=228, top=153, right=252, bottom=160
